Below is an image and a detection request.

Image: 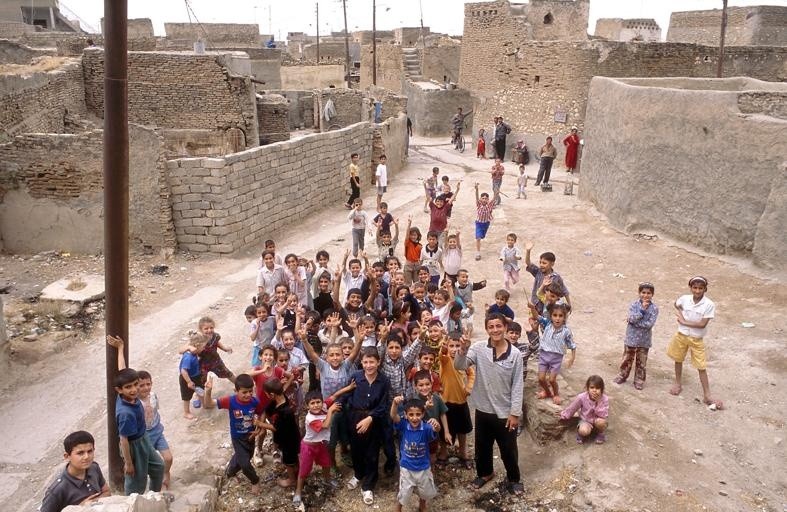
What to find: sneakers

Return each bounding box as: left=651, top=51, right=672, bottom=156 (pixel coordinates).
left=383, top=459, right=396, bottom=472
left=340, top=454, right=353, bottom=467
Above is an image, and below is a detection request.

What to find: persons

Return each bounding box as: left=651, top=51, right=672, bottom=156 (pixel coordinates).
left=188, top=319, right=239, bottom=402
left=104, top=333, right=165, bottom=495
left=133, top=368, right=172, bottom=490
left=489, top=158, right=505, bottom=205
left=436, top=177, right=451, bottom=199
left=265, top=35, right=277, bottom=49
left=511, top=139, right=528, bottom=165
left=447, top=106, right=511, bottom=164
left=406, top=117, right=413, bottom=139
left=665, top=274, right=725, bottom=410
left=373, top=154, right=389, bottom=214
left=514, top=166, right=529, bottom=199
left=472, top=180, right=500, bottom=262
left=177, top=327, right=216, bottom=419
left=561, top=125, right=580, bottom=175
left=204, top=200, right=576, bottom=511
left=532, top=137, right=557, bottom=189
left=343, top=152, right=361, bottom=209
left=422, top=167, right=440, bottom=214
left=559, top=374, right=610, bottom=444
left=424, top=181, right=461, bottom=236
left=39, top=430, right=112, bottom=512
left=614, top=281, right=659, bottom=389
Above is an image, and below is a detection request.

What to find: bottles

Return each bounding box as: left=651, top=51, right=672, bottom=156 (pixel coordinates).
left=193, top=386, right=204, bottom=397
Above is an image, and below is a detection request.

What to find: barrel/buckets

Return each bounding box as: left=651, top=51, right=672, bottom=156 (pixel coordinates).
left=194, top=41, right=204, bottom=53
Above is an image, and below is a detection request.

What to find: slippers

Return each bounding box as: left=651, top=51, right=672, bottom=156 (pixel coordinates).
left=459, top=458, right=473, bottom=470
left=671, top=386, right=682, bottom=395
left=293, top=495, right=301, bottom=506
left=435, top=452, right=448, bottom=470
left=504, top=476, right=524, bottom=494
left=472, top=474, right=495, bottom=488
left=594, top=432, right=605, bottom=444
left=193, top=396, right=200, bottom=407
left=613, top=376, right=625, bottom=384
left=576, top=432, right=585, bottom=444
left=322, top=468, right=374, bottom=505
left=702, top=396, right=723, bottom=410
left=536, top=391, right=562, bottom=404
left=635, top=382, right=643, bottom=389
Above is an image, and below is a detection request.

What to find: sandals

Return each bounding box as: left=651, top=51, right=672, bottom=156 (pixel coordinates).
left=272, top=448, right=283, bottom=467
left=252, top=446, right=264, bottom=468
